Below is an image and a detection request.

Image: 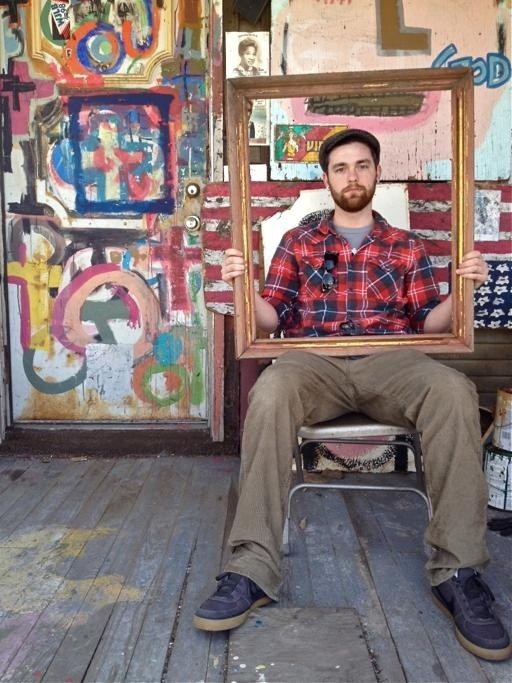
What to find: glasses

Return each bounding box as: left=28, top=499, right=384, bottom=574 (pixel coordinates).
left=321, top=252, right=340, bottom=293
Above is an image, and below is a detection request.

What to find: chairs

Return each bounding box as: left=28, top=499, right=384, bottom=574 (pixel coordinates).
left=257, top=182, right=433, bottom=558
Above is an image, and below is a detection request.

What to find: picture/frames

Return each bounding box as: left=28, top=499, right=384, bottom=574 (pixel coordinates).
left=224, top=65, right=478, bottom=360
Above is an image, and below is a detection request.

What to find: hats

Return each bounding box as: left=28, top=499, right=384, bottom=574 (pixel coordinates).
left=318, top=130, right=381, bottom=171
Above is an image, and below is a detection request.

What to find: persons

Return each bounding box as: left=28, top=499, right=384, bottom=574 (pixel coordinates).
left=280, top=128, right=300, bottom=160
left=192, top=126, right=512, bottom=661
left=229, top=38, right=264, bottom=77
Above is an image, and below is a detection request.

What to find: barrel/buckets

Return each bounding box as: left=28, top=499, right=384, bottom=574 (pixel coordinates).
left=483, top=446, right=512, bottom=511
left=492, top=388, right=512, bottom=455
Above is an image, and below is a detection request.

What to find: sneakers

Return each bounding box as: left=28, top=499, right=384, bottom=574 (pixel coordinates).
left=434, top=570, right=510, bottom=663
left=192, top=575, right=267, bottom=632
